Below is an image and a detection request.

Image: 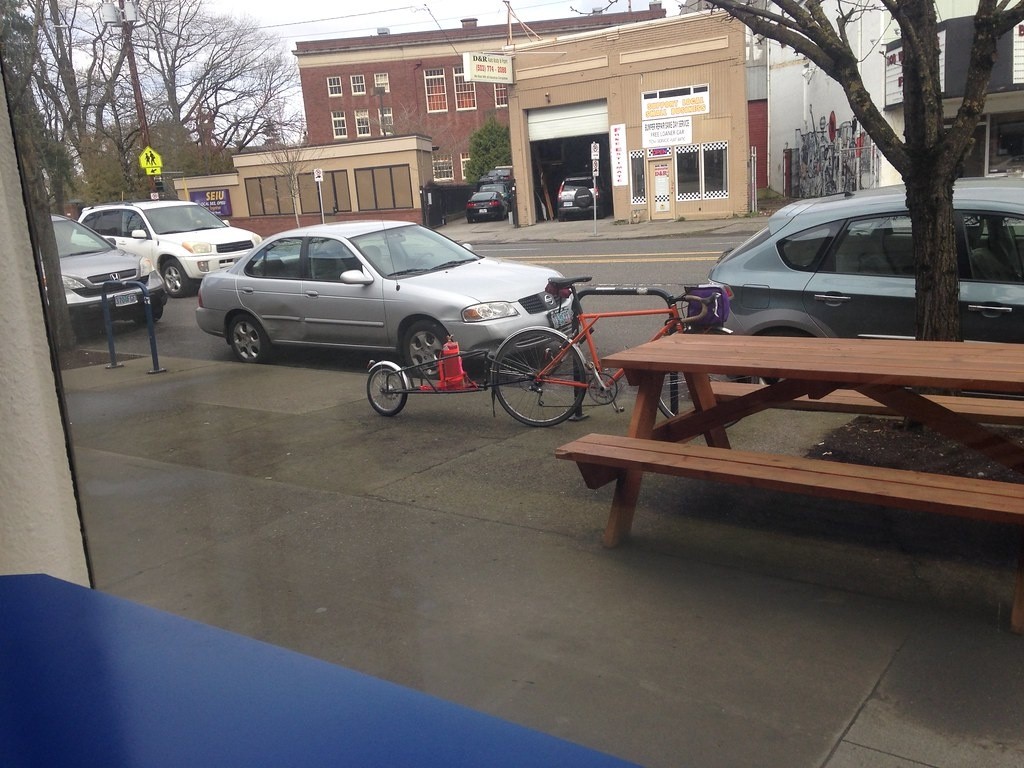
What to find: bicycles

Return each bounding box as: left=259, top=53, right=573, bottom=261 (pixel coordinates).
left=490, top=275, right=759, bottom=429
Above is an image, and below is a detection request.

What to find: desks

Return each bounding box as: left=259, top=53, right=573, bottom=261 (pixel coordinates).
left=600, top=333, right=1024, bottom=632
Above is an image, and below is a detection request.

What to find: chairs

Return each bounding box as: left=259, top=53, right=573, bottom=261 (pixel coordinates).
left=313, top=251, right=337, bottom=280
left=985, top=213, right=1020, bottom=273
left=361, top=245, right=383, bottom=264
left=965, top=215, right=1018, bottom=281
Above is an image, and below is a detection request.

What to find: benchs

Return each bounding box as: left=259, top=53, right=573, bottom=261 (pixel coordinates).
left=708, top=380, right=1024, bottom=427
left=552, top=433, right=1024, bottom=526
left=785, top=233, right=877, bottom=275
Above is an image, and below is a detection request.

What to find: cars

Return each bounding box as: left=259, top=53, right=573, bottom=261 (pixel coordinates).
left=195, top=220, right=575, bottom=380
left=40, top=215, right=167, bottom=336
left=706, top=175, right=1024, bottom=388
left=466, top=192, right=508, bottom=224
left=480, top=184, right=512, bottom=212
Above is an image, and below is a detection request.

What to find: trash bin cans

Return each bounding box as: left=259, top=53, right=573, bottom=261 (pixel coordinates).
left=62, top=202, right=86, bottom=220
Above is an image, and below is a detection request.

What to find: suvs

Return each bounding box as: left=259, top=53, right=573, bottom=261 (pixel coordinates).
left=557, top=176, right=604, bottom=222
left=70, top=199, right=262, bottom=298
